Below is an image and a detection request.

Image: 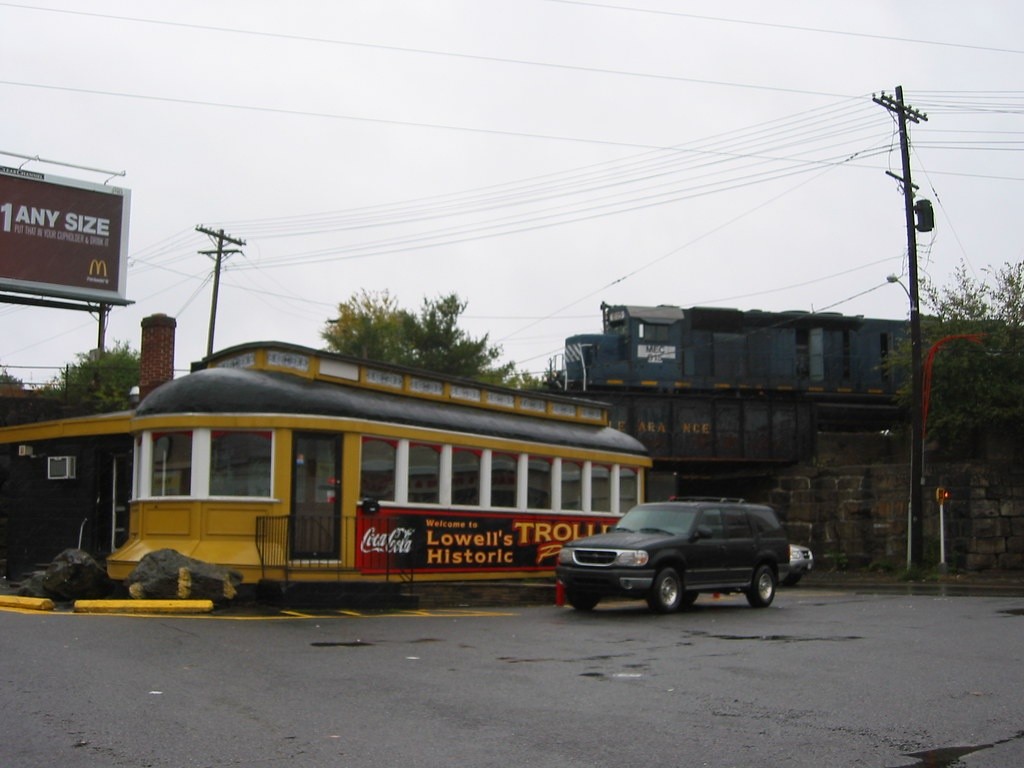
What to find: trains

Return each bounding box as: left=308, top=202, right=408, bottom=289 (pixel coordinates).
left=564, top=301, right=1024, bottom=398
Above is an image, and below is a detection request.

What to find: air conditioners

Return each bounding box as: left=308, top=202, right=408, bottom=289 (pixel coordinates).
left=47, top=456, right=76, bottom=480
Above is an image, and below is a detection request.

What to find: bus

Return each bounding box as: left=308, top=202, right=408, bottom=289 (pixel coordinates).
left=108, top=342, right=653, bottom=603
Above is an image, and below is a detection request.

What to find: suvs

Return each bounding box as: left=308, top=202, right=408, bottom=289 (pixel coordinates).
left=776, top=543, right=812, bottom=589
left=556, top=495, right=791, bottom=613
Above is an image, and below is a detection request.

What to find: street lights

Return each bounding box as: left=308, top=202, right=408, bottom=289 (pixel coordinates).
left=887, top=275, right=924, bottom=578
